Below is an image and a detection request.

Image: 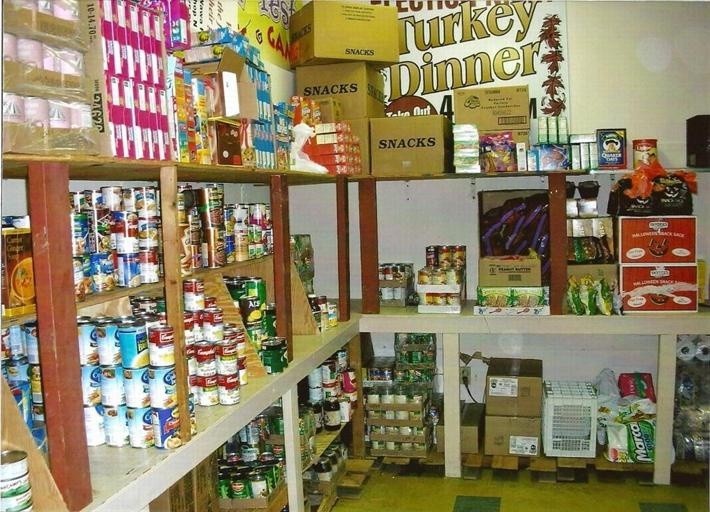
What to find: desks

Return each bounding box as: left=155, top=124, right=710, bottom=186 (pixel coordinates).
left=368, top=167, right=709, bottom=490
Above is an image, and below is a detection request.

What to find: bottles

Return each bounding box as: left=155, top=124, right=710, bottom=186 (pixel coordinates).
left=307, top=293, right=329, bottom=332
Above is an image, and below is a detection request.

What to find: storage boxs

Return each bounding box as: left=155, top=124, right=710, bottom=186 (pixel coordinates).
left=619, top=215, right=697, bottom=264
left=182, top=27, right=364, bottom=176
left=453, top=84, right=531, bottom=137
left=619, top=265, right=698, bottom=314
left=540, top=379, right=598, bottom=458
left=292, top=62, right=386, bottom=176
left=476, top=246, right=542, bottom=287
left=290, top=2, right=399, bottom=70
left=370, top=114, right=454, bottom=176
left=473, top=129, right=532, bottom=174
left=2, top=0, right=114, bottom=158
left=485, top=356, right=545, bottom=417
left=481, top=416, right=545, bottom=458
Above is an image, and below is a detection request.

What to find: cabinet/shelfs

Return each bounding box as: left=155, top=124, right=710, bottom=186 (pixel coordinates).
left=4, top=153, right=366, bottom=512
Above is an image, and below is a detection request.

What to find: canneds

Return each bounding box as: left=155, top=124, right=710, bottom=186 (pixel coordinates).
left=290, top=235, right=339, bottom=330
left=631, top=139, right=657, bottom=171
left=0, top=276, right=290, bottom=510
left=296, top=348, right=357, bottom=510
left=0, top=182, right=273, bottom=317
